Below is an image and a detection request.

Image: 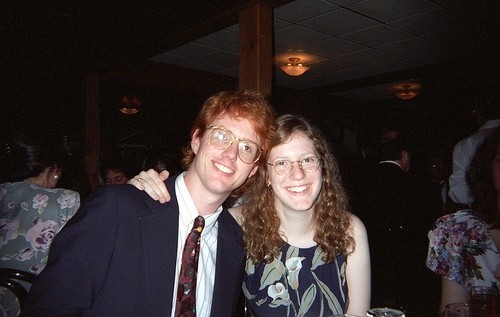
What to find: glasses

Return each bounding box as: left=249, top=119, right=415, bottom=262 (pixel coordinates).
left=264, top=153, right=326, bottom=174
left=203, top=124, right=262, bottom=165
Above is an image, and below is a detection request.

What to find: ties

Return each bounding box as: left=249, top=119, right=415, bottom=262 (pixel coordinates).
left=170, top=215, right=206, bottom=317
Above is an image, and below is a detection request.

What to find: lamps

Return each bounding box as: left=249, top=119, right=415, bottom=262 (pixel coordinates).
left=280, top=58, right=311, bottom=76
left=394, top=85, right=417, bottom=99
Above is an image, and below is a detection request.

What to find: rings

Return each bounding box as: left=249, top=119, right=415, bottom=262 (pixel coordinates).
left=138, top=178, right=143, bottom=182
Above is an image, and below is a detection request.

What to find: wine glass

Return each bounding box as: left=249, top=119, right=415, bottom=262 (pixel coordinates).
left=432, top=164, right=444, bottom=186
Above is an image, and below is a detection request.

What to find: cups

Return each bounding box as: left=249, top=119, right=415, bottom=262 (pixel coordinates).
left=444, top=287, right=500, bottom=317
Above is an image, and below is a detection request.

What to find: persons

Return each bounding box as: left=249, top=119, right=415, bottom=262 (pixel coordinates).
left=127, top=114, right=371, bottom=317
left=23, top=88, right=274, bottom=317
left=101, top=164, right=128, bottom=185
left=363, top=104, right=500, bottom=317
left=0, top=131, right=80, bottom=317
